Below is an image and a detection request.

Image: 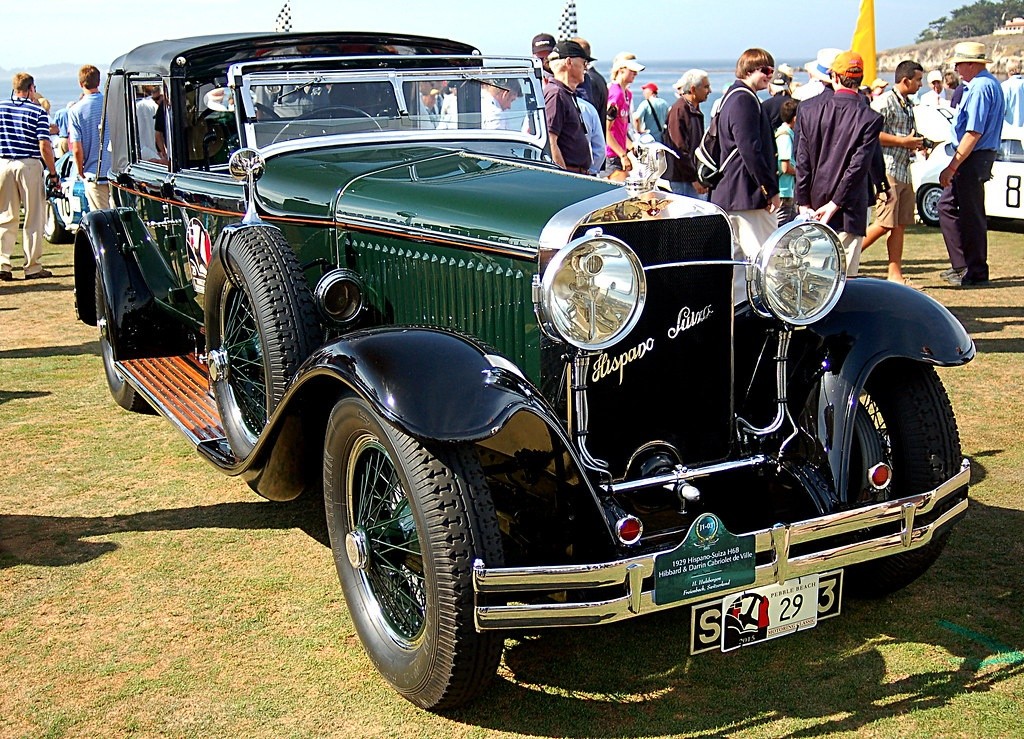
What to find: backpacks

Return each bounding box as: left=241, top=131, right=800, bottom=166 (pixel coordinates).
left=695, top=87, right=762, bottom=190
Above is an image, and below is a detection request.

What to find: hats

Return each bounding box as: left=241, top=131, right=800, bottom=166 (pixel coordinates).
left=532, top=33, right=556, bottom=53
left=805, top=48, right=839, bottom=83
left=871, top=78, right=888, bottom=90
left=549, top=40, right=597, bottom=61
left=642, top=83, right=658, bottom=93
left=770, top=71, right=791, bottom=86
left=826, top=50, right=864, bottom=77
left=927, top=70, right=943, bottom=82
left=946, top=42, right=993, bottom=64
left=613, top=58, right=646, bottom=71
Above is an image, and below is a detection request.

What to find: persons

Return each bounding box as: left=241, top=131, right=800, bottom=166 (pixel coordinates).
left=708, top=41, right=1024, bottom=309
left=136, top=85, right=167, bottom=164
left=33, top=91, right=85, bottom=169
left=0, top=73, right=61, bottom=281
left=68, top=66, right=112, bottom=211
left=415, top=33, right=712, bottom=199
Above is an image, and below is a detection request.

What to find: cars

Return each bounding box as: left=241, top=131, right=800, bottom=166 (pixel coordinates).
left=906, top=103, right=1024, bottom=227
left=75, top=30, right=978, bottom=718
left=41, top=150, right=89, bottom=245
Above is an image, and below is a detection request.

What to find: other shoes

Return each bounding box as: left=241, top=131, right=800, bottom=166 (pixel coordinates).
left=24, top=269, right=52, bottom=279
left=904, top=278, right=926, bottom=291
left=0, top=270, right=12, bottom=280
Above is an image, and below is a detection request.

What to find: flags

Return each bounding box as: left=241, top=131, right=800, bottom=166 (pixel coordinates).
left=557, top=0, right=578, bottom=42
left=276, top=0, right=293, bottom=34
left=850, top=0, right=877, bottom=88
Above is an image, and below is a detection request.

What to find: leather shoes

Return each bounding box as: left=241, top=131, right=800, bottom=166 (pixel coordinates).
left=939, top=267, right=959, bottom=280
left=948, top=269, right=990, bottom=286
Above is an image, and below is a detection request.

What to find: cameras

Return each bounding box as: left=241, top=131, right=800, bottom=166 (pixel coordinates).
left=915, top=132, right=934, bottom=148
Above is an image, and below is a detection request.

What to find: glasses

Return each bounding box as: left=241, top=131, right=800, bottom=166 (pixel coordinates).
left=754, top=65, right=775, bottom=76
left=572, top=59, right=588, bottom=66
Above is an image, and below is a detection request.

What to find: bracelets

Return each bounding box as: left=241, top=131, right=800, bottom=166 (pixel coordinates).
left=949, top=165, right=956, bottom=172
left=49, top=173, right=57, bottom=178
